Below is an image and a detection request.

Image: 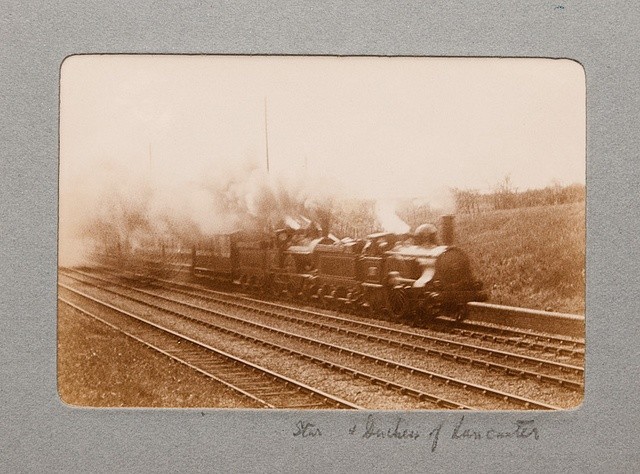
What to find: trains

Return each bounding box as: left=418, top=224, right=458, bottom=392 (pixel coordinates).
left=104, top=216, right=489, bottom=325
